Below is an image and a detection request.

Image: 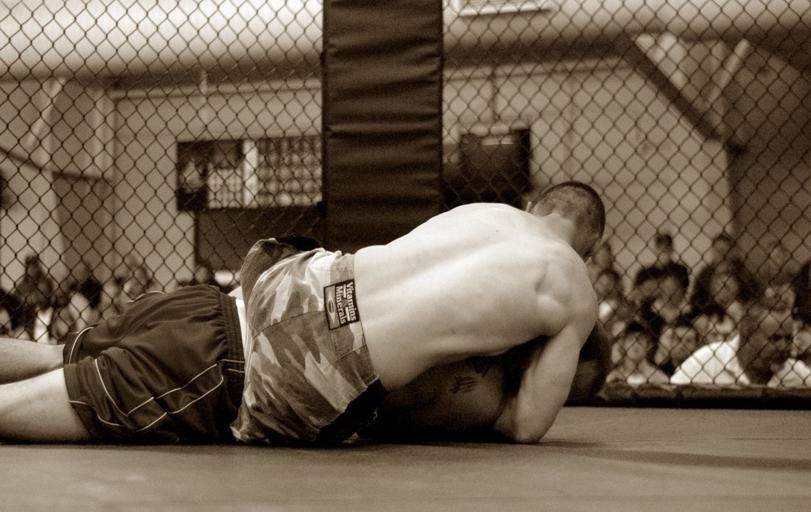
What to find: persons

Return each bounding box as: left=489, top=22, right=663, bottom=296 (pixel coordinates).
left=225, top=181, right=607, bottom=444
left=0, top=254, right=222, bottom=344
left=588, top=228, right=811, bottom=396
left=1, top=285, right=608, bottom=445
